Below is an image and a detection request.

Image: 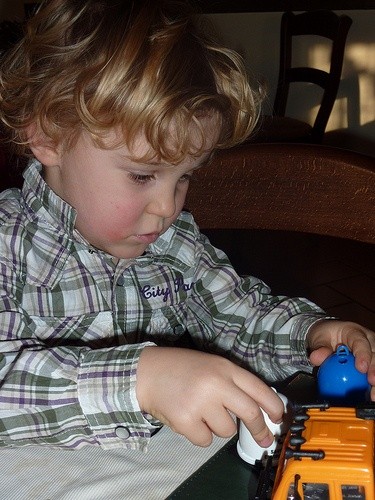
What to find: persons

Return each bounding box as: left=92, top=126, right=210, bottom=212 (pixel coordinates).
left=0, top=0, right=375, bottom=454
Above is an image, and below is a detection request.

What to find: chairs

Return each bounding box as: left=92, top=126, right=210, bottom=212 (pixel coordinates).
left=249, top=11, right=353, bottom=140
left=184, top=144, right=375, bottom=246
